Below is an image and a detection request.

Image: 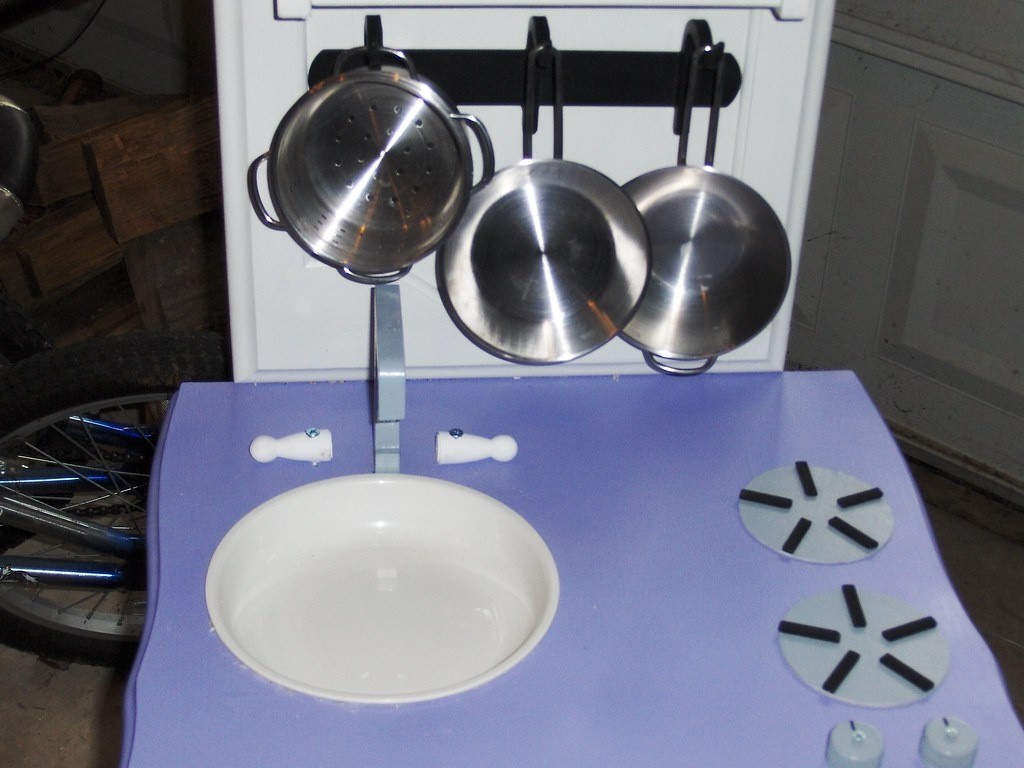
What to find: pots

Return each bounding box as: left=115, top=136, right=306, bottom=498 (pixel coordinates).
left=436, top=19, right=652, bottom=366
left=616, top=43, right=792, bottom=380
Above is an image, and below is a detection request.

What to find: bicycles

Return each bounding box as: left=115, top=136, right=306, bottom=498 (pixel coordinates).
left=1, top=95, right=234, bottom=665
